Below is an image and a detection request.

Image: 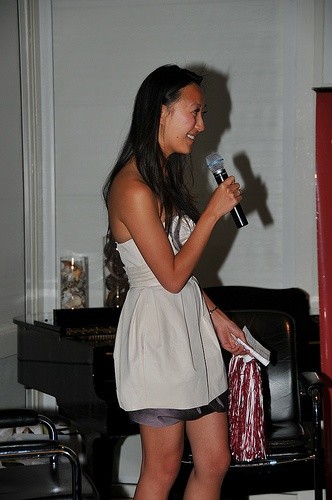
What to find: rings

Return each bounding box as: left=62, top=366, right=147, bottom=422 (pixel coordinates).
left=236, top=189, right=241, bottom=195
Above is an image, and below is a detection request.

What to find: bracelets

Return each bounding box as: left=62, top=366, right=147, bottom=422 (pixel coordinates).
left=208, top=305, right=219, bottom=315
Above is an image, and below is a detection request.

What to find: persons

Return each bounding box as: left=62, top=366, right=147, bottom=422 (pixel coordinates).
left=103, top=65, right=243, bottom=500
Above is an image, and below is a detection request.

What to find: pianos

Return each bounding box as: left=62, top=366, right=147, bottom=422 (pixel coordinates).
left=13, top=279, right=332, bottom=499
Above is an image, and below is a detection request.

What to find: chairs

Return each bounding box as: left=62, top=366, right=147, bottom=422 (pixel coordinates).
left=1, top=408, right=83, bottom=500
left=169, top=307, right=332, bottom=500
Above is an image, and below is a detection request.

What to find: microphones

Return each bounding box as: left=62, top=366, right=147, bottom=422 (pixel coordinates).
left=206, top=152, right=248, bottom=229
